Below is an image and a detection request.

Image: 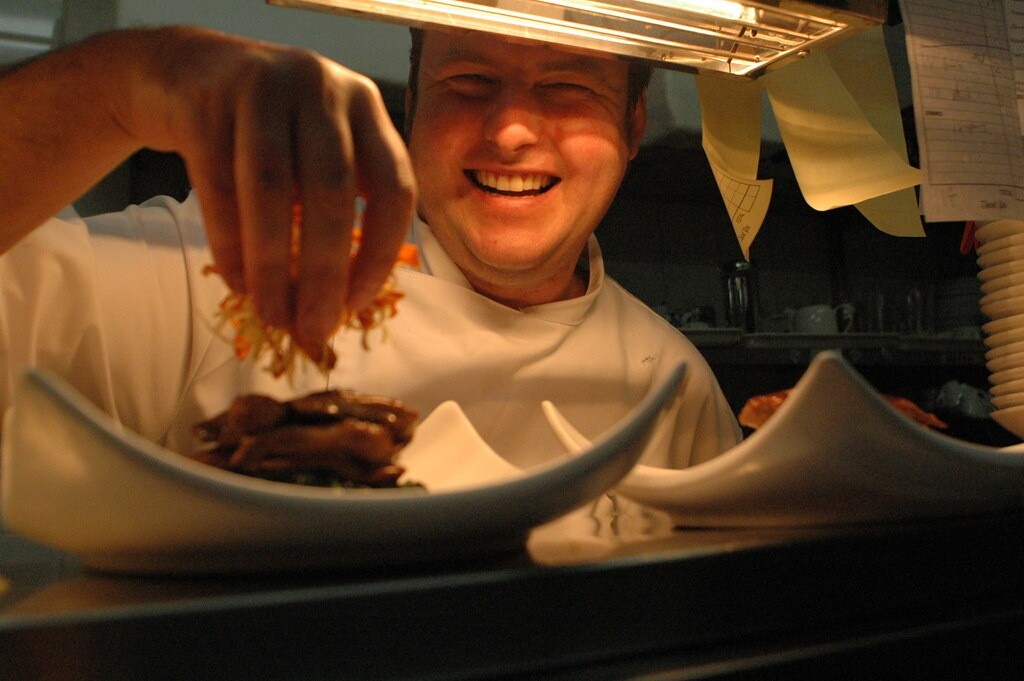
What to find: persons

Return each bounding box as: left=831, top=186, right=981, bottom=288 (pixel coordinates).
left=0, top=25, right=745, bottom=541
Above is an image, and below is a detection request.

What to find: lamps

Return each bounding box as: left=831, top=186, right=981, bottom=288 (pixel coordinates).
left=264, top=0, right=889, bottom=79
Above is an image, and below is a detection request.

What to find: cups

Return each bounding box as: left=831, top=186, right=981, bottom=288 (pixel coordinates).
left=790, top=304, right=838, bottom=335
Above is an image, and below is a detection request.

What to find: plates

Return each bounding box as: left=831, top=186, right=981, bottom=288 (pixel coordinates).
left=539, top=346, right=1023, bottom=533
left=1, top=356, right=694, bottom=574
left=975, top=219, right=1024, bottom=447
